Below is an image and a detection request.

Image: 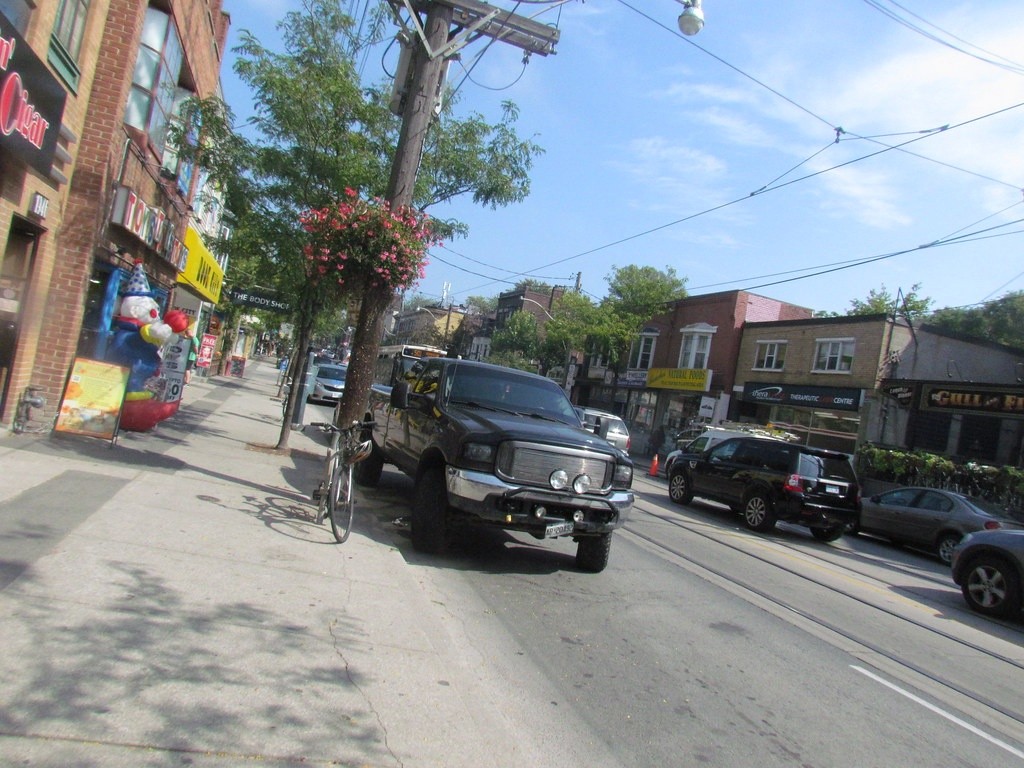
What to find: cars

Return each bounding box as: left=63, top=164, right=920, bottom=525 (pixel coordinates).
left=861, top=487, right=1024, bottom=619
left=307, top=355, right=348, bottom=402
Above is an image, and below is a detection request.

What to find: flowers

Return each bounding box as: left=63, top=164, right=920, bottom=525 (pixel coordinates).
left=299, top=186, right=445, bottom=306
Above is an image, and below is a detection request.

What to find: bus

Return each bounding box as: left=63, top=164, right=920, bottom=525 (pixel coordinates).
left=374, top=344, right=448, bottom=387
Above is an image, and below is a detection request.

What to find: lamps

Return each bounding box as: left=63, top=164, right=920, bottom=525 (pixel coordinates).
left=185, top=212, right=201, bottom=224
left=947, top=360, right=964, bottom=382
left=145, top=160, right=177, bottom=182
left=1015, top=363, right=1024, bottom=384
left=160, top=182, right=185, bottom=197
left=170, top=199, right=194, bottom=212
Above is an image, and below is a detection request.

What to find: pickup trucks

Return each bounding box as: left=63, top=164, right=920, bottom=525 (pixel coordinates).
left=364, top=357, right=635, bottom=574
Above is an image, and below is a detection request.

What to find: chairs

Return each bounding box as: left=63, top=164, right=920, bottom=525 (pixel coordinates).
left=744, top=447, right=760, bottom=459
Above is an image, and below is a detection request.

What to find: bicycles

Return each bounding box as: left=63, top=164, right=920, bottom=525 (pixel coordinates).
left=283, top=374, right=295, bottom=420
left=309, top=422, right=379, bottom=543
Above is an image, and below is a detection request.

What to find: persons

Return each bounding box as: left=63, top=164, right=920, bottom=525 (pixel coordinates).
left=185, top=329, right=199, bottom=384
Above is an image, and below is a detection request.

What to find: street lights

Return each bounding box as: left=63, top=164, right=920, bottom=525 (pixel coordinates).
left=518, top=296, right=569, bottom=389
left=415, top=306, right=455, bottom=351
left=336, top=326, right=352, bottom=346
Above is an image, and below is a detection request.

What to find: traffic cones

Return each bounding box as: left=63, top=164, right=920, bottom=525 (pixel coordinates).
left=645, top=454, right=664, bottom=478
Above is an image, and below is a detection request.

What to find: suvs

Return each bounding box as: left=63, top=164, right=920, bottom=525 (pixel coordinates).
left=666, top=429, right=864, bottom=543
left=562, top=405, right=633, bottom=451
